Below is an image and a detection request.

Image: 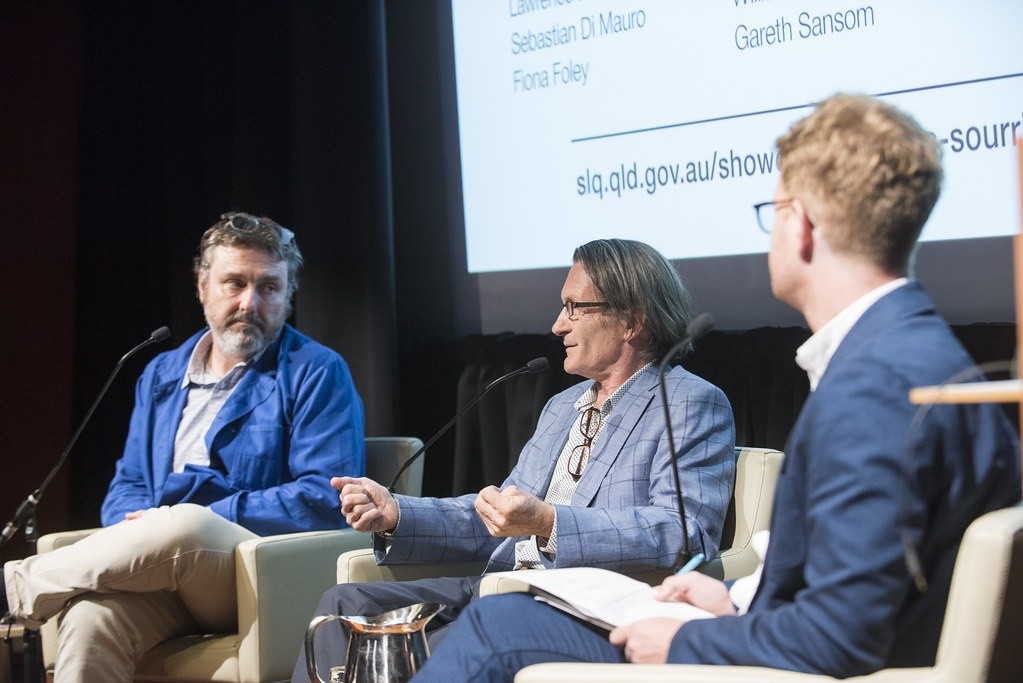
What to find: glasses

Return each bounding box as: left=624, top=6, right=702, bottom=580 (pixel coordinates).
left=224, top=214, right=296, bottom=246
left=564, top=300, right=611, bottom=318
left=754, top=197, right=796, bottom=234
left=568, top=406, right=602, bottom=483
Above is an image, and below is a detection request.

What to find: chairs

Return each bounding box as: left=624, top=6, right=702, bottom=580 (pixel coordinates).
left=337, top=447, right=786, bottom=683
left=36, top=437, right=425, bottom=683
left=513, top=505, right=1023, bottom=683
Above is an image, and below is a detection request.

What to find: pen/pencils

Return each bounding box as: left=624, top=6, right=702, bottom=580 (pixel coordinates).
left=676, top=552, right=705, bottom=575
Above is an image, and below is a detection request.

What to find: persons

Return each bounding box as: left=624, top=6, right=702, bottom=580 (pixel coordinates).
left=0, top=206, right=370, bottom=683
left=408, top=93, right=1023, bottom=683
left=290, top=238, right=736, bottom=683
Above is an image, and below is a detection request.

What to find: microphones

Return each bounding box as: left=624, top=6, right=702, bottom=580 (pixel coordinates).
left=13, top=326, right=170, bottom=527
left=660, top=312, right=715, bottom=567
left=386, top=355, right=549, bottom=493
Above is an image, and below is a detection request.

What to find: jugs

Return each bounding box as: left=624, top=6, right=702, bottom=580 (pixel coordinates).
left=305, top=600, right=447, bottom=683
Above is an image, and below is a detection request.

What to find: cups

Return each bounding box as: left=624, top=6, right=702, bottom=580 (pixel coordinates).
left=328, top=665, right=346, bottom=683
